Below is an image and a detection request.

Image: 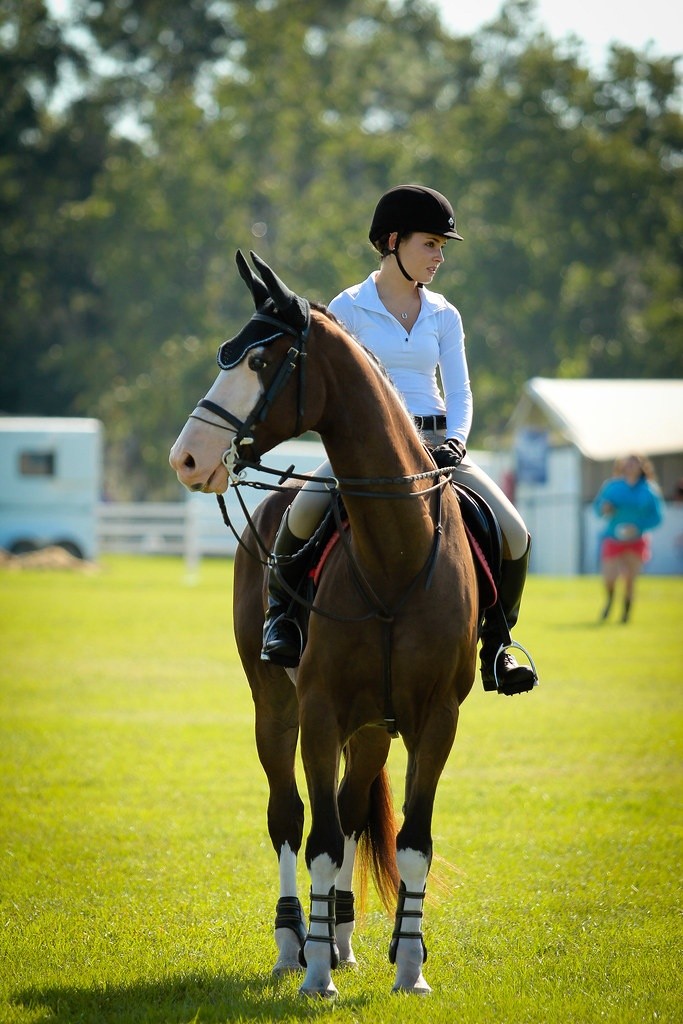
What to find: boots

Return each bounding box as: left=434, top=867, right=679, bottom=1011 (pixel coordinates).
left=479, top=532, right=535, bottom=691
left=259, top=505, right=313, bottom=668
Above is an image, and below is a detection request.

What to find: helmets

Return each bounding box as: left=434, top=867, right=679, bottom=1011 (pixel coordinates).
left=369, top=185, right=463, bottom=253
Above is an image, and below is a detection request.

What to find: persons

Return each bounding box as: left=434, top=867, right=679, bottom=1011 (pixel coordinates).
left=260, top=185, right=538, bottom=694
left=592, top=448, right=665, bottom=625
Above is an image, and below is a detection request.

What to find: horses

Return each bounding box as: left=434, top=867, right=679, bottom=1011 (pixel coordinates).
left=167, top=249, right=482, bottom=998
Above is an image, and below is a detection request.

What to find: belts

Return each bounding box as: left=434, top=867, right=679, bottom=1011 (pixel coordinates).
left=414, top=415, right=447, bottom=431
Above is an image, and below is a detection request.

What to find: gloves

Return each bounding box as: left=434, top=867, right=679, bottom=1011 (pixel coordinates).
left=429, top=439, right=466, bottom=476
